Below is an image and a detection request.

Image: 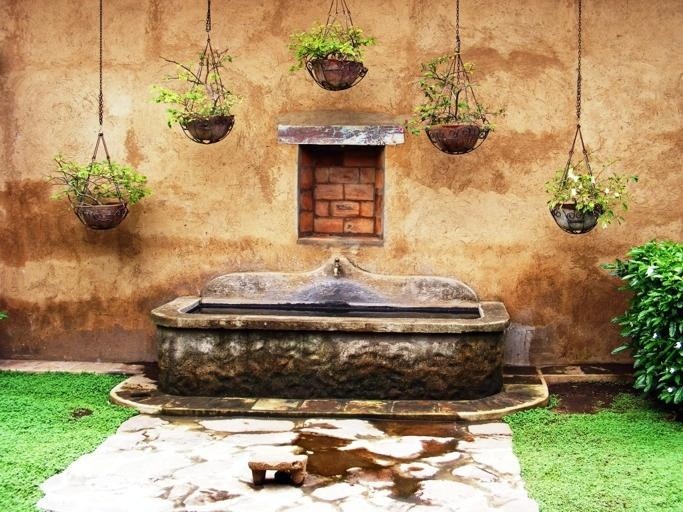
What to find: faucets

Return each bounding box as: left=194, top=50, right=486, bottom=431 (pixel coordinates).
left=333, top=258, right=342, bottom=276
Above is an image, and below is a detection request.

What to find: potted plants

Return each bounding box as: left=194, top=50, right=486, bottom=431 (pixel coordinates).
left=288, top=20, right=379, bottom=91
left=405, top=49, right=507, bottom=154
left=545, top=143, right=640, bottom=235
left=43, top=150, right=153, bottom=231
left=147, top=46, right=245, bottom=145
